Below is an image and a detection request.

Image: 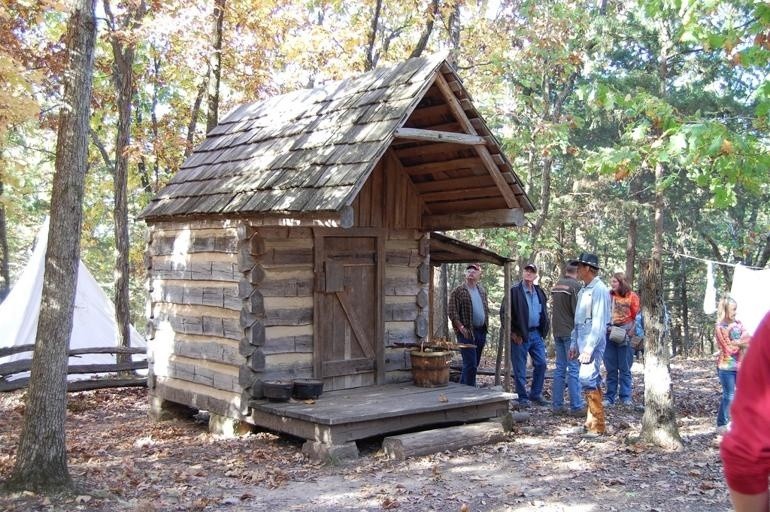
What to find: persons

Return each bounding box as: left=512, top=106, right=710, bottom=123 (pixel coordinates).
left=549, top=259, right=587, bottom=417
left=500, top=264, right=549, bottom=408
left=713, top=294, right=752, bottom=447
left=721, top=311, right=770, bottom=511
left=568, top=254, right=613, bottom=433
left=599, top=272, right=641, bottom=413
left=449, top=265, right=491, bottom=386
left=635, top=290, right=644, bottom=361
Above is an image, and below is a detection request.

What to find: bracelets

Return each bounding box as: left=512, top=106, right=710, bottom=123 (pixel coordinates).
left=459, top=324, right=464, bottom=330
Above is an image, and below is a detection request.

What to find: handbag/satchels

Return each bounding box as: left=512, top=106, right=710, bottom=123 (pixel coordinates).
left=609, top=324, right=626, bottom=344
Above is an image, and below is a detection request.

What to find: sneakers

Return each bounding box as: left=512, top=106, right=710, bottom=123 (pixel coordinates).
left=513, top=392, right=636, bottom=440
left=714, top=423, right=732, bottom=434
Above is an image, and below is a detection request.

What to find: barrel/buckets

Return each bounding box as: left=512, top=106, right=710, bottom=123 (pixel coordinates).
left=408, top=349, right=452, bottom=388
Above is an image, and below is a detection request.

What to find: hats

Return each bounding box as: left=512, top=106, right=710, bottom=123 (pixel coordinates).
left=466, top=264, right=480, bottom=270
left=524, top=263, right=537, bottom=273
left=569, top=252, right=602, bottom=270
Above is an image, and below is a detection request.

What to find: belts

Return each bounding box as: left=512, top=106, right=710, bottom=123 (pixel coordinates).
left=528, top=326, right=539, bottom=331
